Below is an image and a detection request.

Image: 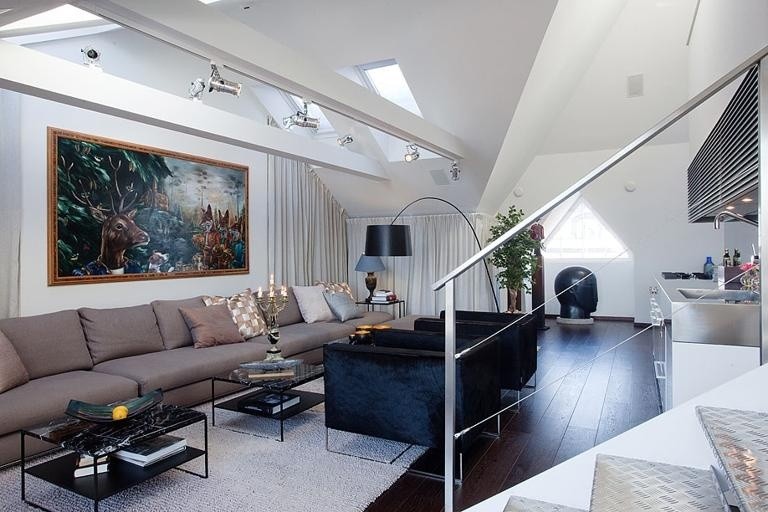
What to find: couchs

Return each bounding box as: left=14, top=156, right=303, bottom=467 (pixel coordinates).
left=1, top=288, right=392, bottom=472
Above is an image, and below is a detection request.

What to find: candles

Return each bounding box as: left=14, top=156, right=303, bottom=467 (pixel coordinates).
left=257, top=275, right=288, bottom=298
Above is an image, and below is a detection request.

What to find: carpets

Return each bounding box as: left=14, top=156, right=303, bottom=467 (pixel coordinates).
left=0, top=375, right=427, bottom=512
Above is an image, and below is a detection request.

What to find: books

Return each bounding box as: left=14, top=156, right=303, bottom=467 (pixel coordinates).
left=237, top=389, right=300, bottom=414
left=111, top=446, right=186, bottom=467
left=73, top=452, right=109, bottom=478
left=371, top=289, right=400, bottom=304
left=109, top=433, right=187, bottom=463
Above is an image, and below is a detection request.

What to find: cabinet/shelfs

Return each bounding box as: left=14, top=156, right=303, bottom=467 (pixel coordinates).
left=649, top=291, right=762, bottom=414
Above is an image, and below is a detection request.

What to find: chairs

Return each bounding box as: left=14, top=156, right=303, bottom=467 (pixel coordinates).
left=414, top=309, right=537, bottom=413
left=323, top=329, right=500, bottom=486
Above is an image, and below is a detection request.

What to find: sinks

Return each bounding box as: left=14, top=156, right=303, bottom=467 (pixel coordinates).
left=679, top=288, right=760, bottom=302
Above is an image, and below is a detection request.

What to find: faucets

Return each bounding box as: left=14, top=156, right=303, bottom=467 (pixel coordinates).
left=713, top=211, right=758, bottom=231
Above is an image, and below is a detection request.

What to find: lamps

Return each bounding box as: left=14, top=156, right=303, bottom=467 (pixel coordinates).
left=81, top=45, right=461, bottom=180
left=365, top=197, right=500, bottom=313
left=355, top=253, right=384, bottom=302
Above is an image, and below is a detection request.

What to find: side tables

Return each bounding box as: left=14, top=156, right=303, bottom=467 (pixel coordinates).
left=355, top=299, right=405, bottom=321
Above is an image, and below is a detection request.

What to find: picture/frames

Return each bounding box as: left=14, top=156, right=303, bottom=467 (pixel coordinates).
left=48, top=128, right=250, bottom=286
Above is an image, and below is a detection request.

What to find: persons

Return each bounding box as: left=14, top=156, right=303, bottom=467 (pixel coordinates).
left=554, top=266, right=598, bottom=324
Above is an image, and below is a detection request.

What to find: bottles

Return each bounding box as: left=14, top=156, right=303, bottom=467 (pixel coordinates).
left=703, top=256, right=714, bottom=276
left=722, top=248, right=730, bottom=266
left=733, top=248, right=742, bottom=265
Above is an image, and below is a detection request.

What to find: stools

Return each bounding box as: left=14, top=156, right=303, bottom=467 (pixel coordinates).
left=386, top=313, right=440, bottom=331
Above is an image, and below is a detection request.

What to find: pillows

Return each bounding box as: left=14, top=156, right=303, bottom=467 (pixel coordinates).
left=179, top=301, right=245, bottom=349
left=200, top=289, right=268, bottom=339
left=254, top=287, right=304, bottom=330
left=151, top=296, right=206, bottom=353
left=78, top=304, right=165, bottom=365
left=291, top=283, right=339, bottom=323
left=322, top=283, right=363, bottom=323
left=2, top=330, right=32, bottom=392
left=2, top=309, right=94, bottom=380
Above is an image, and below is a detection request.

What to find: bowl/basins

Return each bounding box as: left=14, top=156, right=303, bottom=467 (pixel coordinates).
left=348, top=324, right=392, bottom=342
